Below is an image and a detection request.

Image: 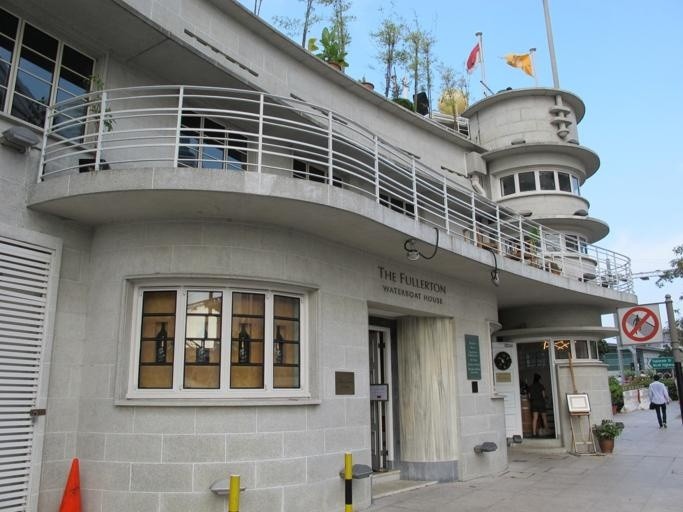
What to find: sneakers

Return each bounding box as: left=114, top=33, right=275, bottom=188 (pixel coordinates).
left=659, top=423, right=667, bottom=429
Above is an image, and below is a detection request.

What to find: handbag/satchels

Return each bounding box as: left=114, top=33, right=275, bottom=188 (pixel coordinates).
left=650, top=402, right=655, bottom=410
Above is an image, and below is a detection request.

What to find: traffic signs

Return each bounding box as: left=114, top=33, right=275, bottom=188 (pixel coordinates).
left=646, top=356, right=676, bottom=370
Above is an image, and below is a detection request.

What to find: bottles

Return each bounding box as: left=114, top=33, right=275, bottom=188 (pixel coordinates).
left=154, top=320, right=284, bottom=366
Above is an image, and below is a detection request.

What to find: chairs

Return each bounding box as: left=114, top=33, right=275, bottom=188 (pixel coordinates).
left=504, top=239, right=536, bottom=268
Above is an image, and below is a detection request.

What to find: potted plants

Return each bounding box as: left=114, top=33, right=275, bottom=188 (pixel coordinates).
left=608, top=376, right=623, bottom=415
left=358, top=76, right=375, bottom=91
left=76, top=72, right=116, bottom=172
left=591, top=419, right=624, bottom=453
left=308, top=26, right=352, bottom=74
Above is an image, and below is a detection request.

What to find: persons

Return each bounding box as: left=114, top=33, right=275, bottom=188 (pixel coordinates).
left=527, top=373, right=548, bottom=436
left=648, top=375, right=670, bottom=428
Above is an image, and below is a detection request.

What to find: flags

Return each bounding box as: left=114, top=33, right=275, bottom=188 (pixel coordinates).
left=463, top=44, right=480, bottom=74
left=501, top=52, right=533, bottom=77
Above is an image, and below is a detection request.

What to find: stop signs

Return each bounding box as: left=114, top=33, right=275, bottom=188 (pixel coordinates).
left=616, top=304, right=665, bottom=347
